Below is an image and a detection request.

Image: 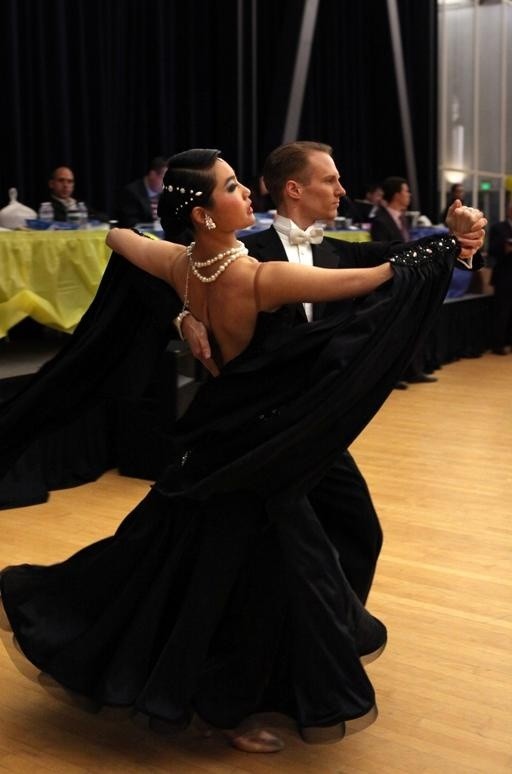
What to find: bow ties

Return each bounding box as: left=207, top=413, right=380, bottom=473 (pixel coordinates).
left=287, top=223, right=325, bottom=247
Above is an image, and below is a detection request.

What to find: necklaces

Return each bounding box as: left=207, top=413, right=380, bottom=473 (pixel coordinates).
left=186, top=238, right=250, bottom=284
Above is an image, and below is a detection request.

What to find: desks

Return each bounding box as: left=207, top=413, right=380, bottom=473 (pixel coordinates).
left=0, top=225, right=471, bottom=330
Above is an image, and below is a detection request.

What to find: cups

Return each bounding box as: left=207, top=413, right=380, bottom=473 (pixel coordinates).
left=333, top=216, right=345, bottom=229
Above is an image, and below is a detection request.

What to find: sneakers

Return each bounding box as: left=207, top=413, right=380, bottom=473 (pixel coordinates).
left=393, top=379, right=408, bottom=390
left=407, top=374, right=438, bottom=383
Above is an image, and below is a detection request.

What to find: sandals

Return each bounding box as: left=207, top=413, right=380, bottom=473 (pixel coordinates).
left=198, top=716, right=286, bottom=752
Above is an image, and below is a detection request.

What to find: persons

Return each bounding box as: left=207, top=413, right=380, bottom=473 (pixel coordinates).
left=438, top=183, right=469, bottom=226
left=1, top=148, right=486, bottom=756
left=369, top=176, right=438, bottom=390
left=119, top=155, right=171, bottom=228
left=248, top=172, right=276, bottom=211
left=354, top=186, right=385, bottom=222
left=486, top=196, right=512, bottom=356
left=159, top=140, right=490, bottom=606
left=38, top=166, right=111, bottom=223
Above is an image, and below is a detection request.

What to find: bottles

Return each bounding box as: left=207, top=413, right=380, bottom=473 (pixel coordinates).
left=38, top=202, right=88, bottom=231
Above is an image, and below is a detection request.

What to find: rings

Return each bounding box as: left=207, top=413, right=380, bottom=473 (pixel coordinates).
left=466, top=207, right=474, bottom=212
